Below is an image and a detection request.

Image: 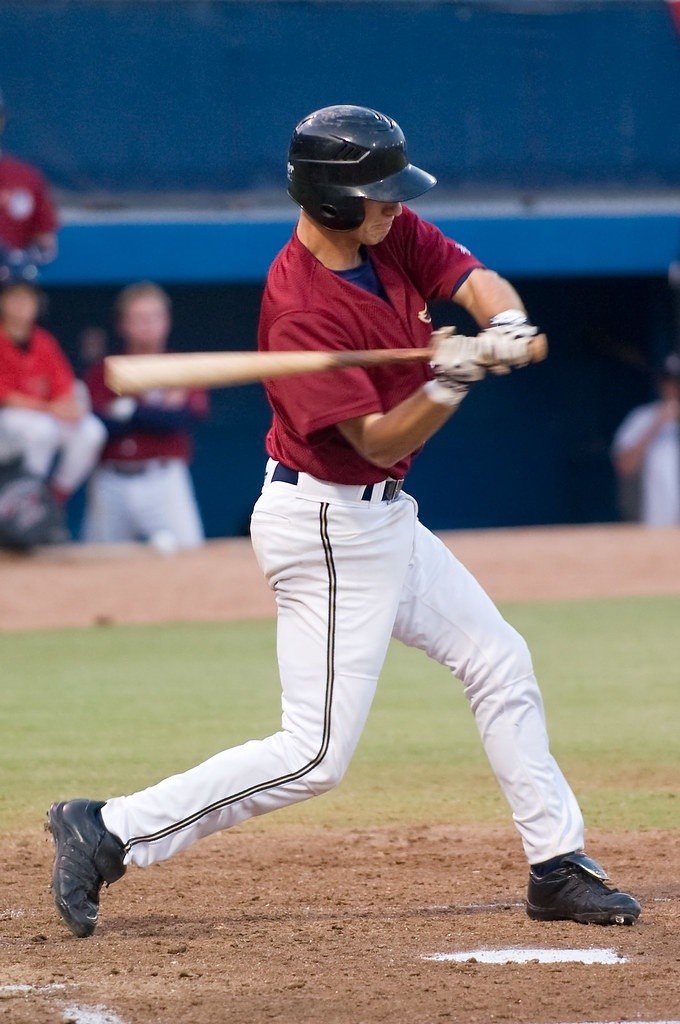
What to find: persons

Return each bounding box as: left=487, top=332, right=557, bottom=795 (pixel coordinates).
left=48, top=104, right=643, bottom=936
left=0, top=148, right=680, bottom=549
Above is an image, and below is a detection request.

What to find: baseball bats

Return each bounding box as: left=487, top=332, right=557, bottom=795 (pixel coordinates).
left=101, top=331, right=552, bottom=395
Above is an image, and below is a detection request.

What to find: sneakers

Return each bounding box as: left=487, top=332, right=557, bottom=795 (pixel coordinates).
left=525, top=853, right=641, bottom=925
left=49, top=798, right=131, bottom=938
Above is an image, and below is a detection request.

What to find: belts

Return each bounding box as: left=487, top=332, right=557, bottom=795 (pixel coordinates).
left=106, top=458, right=170, bottom=475
left=273, top=462, right=404, bottom=503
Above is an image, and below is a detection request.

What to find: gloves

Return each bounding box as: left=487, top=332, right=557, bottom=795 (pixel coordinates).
left=477, top=309, right=540, bottom=374
left=423, top=324, right=487, bottom=404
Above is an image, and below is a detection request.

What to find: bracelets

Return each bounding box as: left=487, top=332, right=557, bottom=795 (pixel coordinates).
left=491, top=308, right=526, bottom=322
left=425, top=381, right=466, bottom=407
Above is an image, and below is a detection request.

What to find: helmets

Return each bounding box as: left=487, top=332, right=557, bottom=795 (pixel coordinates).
left=286, top=105, right=436, bottom=231
